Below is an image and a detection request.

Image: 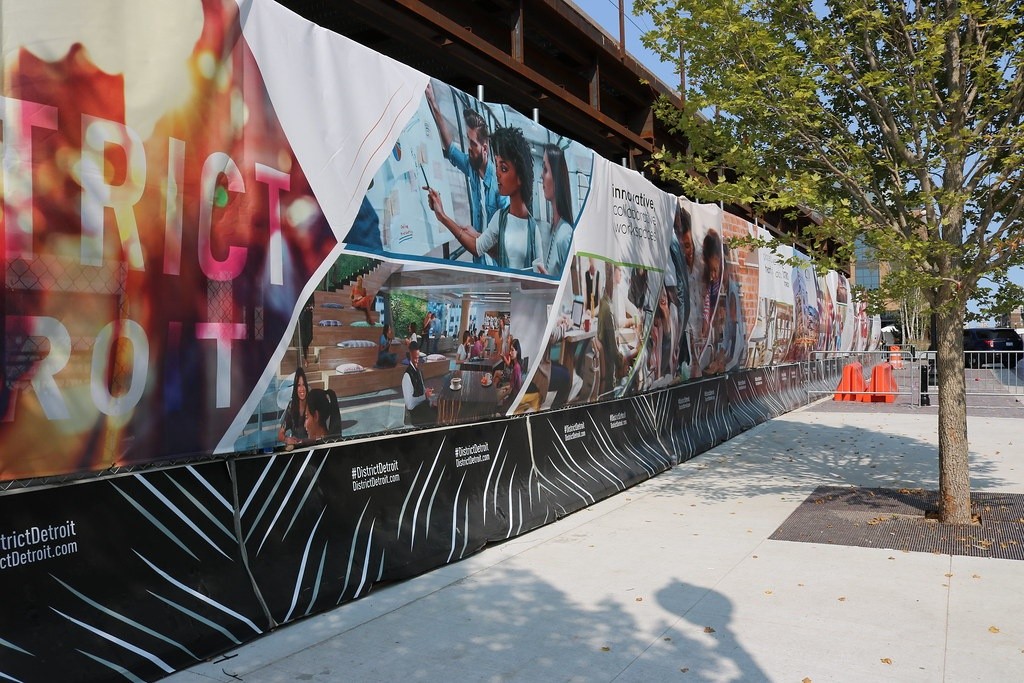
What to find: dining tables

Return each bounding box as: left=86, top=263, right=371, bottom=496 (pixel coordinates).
left=456, top=354, right=504, bottom=369
left=437, top=369, right=497, bottom=425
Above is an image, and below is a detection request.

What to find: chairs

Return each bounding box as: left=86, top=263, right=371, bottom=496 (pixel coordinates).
left=274, top=371, right=312, bottom=444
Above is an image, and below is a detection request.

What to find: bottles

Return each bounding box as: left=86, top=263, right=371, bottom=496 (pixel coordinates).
left=484, top=351, right=487, bottom=358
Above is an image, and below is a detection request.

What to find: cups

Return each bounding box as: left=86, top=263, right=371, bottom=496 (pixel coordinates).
left=583, top=319, right=590, bottom=332
left=485, top=373, right=492, bottom=380
left=451, top=378, right=461, bottom=386
left=474, top=357, right=477, bottom=359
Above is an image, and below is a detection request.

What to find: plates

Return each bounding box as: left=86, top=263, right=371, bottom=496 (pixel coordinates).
left=450, top=385, right=461, bottom=390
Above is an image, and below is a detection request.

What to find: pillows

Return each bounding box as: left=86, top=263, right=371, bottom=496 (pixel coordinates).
left=350, top=321, right=384, bottom=326
left=336, top=339, right=376, bottom=348
left=336, top=363, right=364, bottom=371
left=427, top=353, right=446, bottom=360
left=318, top=319, right=343, bottom=327
left=320, top=302, right=344, bottom=308
left=400, top=356, right=425, bottom=364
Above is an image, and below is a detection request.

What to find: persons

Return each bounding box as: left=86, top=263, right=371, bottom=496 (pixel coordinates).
left=280, top=82, right=742, bottom=445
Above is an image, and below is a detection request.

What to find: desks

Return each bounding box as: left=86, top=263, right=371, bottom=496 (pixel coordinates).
left=562, top=312, right=597, bottom=385
left=416, top=335, right=453, bottom=353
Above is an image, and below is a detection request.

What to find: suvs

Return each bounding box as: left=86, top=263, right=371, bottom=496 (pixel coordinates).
left=963, top=328, right=1024, bottom=369
